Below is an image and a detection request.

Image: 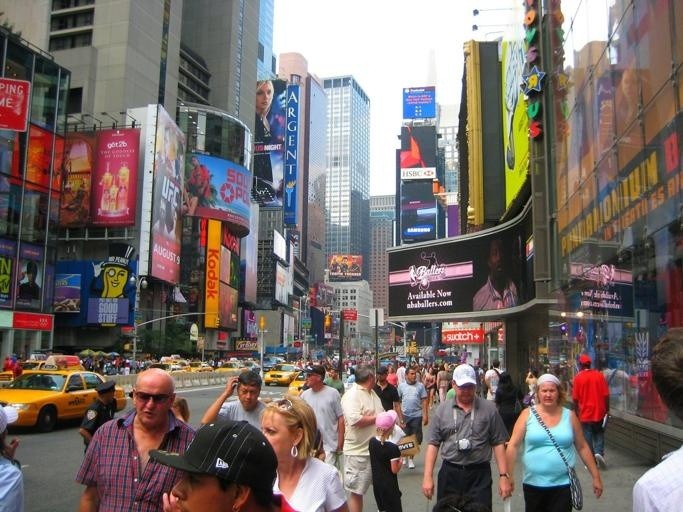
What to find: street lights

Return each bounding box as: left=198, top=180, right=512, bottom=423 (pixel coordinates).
left=130, top=274, right=147, bottom=360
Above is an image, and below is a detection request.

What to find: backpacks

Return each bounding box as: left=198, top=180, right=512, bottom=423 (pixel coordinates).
left=568, top=467, right=583, bottom=510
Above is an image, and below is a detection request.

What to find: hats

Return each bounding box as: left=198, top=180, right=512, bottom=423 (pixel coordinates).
left=376, top=410, right=398, bottom=430
left=149, top=421, right=278, bottom=490
left=0, top=404, right=18, bottom=434
left=96, top=380, right=115, bottom=395
left=302, top=365, right=325, bottom=375
left=376, top=366, right=388, bottom=374
left=453, top=364, right=476, bottom=386
left=579, top=355, right=591, bottom=364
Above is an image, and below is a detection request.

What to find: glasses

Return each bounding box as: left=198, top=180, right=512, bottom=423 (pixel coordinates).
left=273, top=398, right=297, bottom=415
left=135, top=392, right=171, bottom=402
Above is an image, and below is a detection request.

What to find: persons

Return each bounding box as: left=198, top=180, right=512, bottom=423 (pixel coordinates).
left=422, top=362, right=428, bottom=375
left=396, top=362, right=407, bottom=385
left=571, top=354, right=611, bottom=471
left=99, top=359, right=106, bottom=374
left=17, top=261, right=40, bottom=300
left=431, top=361, right=437, bottom=368
left=485, top=360, right=505, bottom=401
left=12, top=357, right=23, bottom=381
left=422, top=367, right=436, bottom=410
left=368, top=409, right=404, bottom=512
left=124, top=359, right=130, bottom=376
left=439, top=359, right=446, bottom=371
left=183, top=157, right=209, bottom=215
left=324, top=368, right=345, bottom=396
left=144, top=361, right=148, bottom=369
left=259, top=395, right=349, bottom=512
left=138, top=360, right=141, bottom=369
left=148, top=358, right=153, bottom=369
left=199, top=371, right=268, bottom=432
left=339, top=258, right=349, bottom=273
left=437, top=364, right=450, bottom=403
left=420, top=364, right=511, bottom=512
left=455, top=360, right=462, bottom=368
left=170, top=397, right=190, bottom=424
left=505, top=374, right=603, bottom=512
left=207, top=359, right=210, bottom=366
left=78, top=380, right=117, bottom=459
left=290, top=355, right=372, bottom=366
left=95, top=359, right=100, bottom=372
left=349, top=257, right=360, bottom=273
left=112, top=360, right=116, bottom=374
left=472, top=240, right=519, bottom=312
left=214, top=359, right=218, bottom=366
left=80, top=358, right=94, bottom=368
left=476, top=361, right=484, bottom=381
left=116, top=357, right=121, bottom=375
left=148, top=420, right=301, bottom=512
left=210, top=359, right=214, bottom=366
left=0, top=404, right=25, bottom=512
left=495, top=371, right=523, bottom=437
left=430, top=364, right=440, bottom=403
left=397, top=367, right=429, bottom=469
left=340, top=367, right=389, bottom=512
left=525, top=369, right=540, bottom=406
left=329, top=256, right=339, bottom=273
left=372, top=366, right=406, bottom=430
left=255, top=81, right=273, bottom=143
left=3, top=354, right=13, bottom=372
left=141, top=360, right=144, bottom=369
left=107, top=360, right=112, bottom=374
left=135, top=359, right=138, bottom=370
left=632, top=327, right=683, bottom=512
left=444, top=362, right=453, bottom=372
left=121, top=359, right=124, bottom=375
left=299, top=367, right=345, bottom=471
left=74, top=368, right=197, bottom=512
left=387, top=367, right=397, bottom=388
left=413, top=364, right=420, bottom=382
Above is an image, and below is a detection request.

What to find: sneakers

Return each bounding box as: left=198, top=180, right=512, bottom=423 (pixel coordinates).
left=402, top=456, right=407, bottom=465
left=408, top=459, right=415, bottom=469
left=595, top=453, right=606, bottom=467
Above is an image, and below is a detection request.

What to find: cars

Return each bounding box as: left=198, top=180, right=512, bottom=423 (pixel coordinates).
left=189, top=362, right=212, bottom=373
left=138, top=355, right=191, bottom=374
left=239, top=358, right=260, bottom=371
left=289, top=371, right=306, bottom=395
left=0, top=356, right=127, bottom=433
left=215, top=358, right=248, bottom=373
left=0, top=360, right=47, bottom=382
left=264, top=360, right=300, bottom=386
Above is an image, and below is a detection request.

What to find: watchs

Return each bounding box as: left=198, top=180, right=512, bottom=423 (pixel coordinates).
left=499, top=473, right=510, bottom=478
